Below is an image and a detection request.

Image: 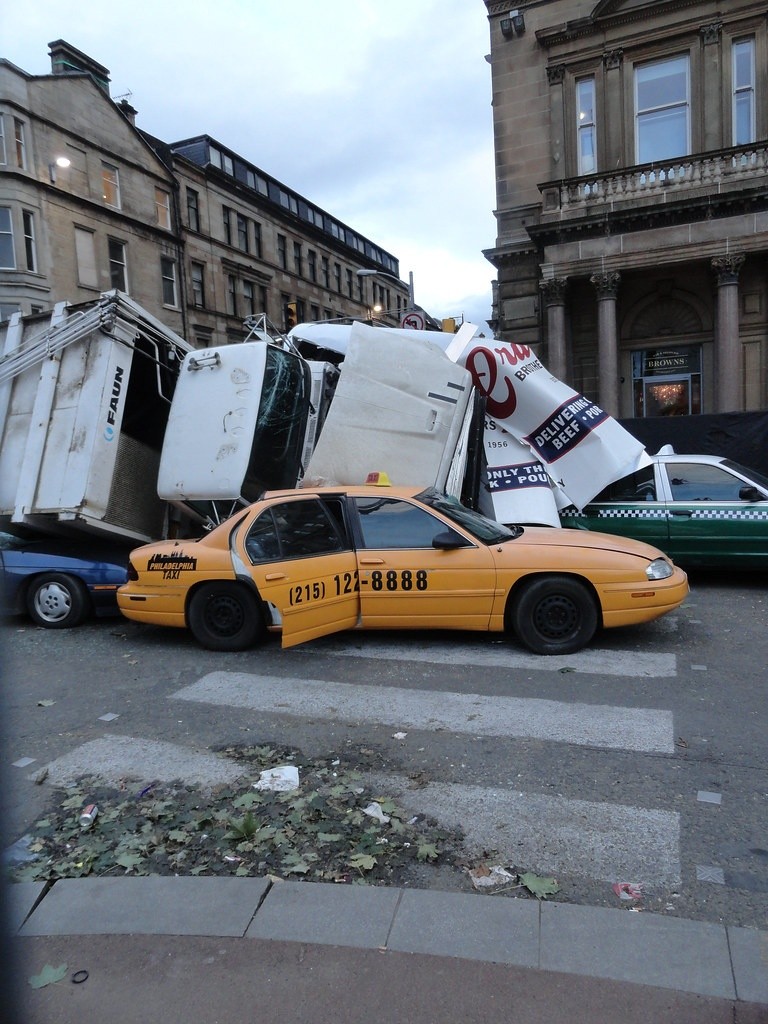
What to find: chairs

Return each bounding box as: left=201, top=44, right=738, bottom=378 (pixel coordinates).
left=246, top=534, right=280, bottom=559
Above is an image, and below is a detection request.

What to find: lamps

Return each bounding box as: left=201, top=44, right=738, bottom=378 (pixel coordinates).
left=500, top=9, right=526, bottom=36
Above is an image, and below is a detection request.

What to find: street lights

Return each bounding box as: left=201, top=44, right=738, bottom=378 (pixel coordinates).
left=357, top=270, right=414, bottom=312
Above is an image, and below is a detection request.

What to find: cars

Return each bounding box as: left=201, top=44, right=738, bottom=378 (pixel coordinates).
left=558, top=444, right=768, bottom=569
left=115, top=472, right=689, bottom=656
left=1, top=532, right=133, bottom=629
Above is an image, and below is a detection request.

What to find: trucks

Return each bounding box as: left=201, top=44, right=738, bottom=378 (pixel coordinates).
left=0, top=287, right=488, bottom=545
